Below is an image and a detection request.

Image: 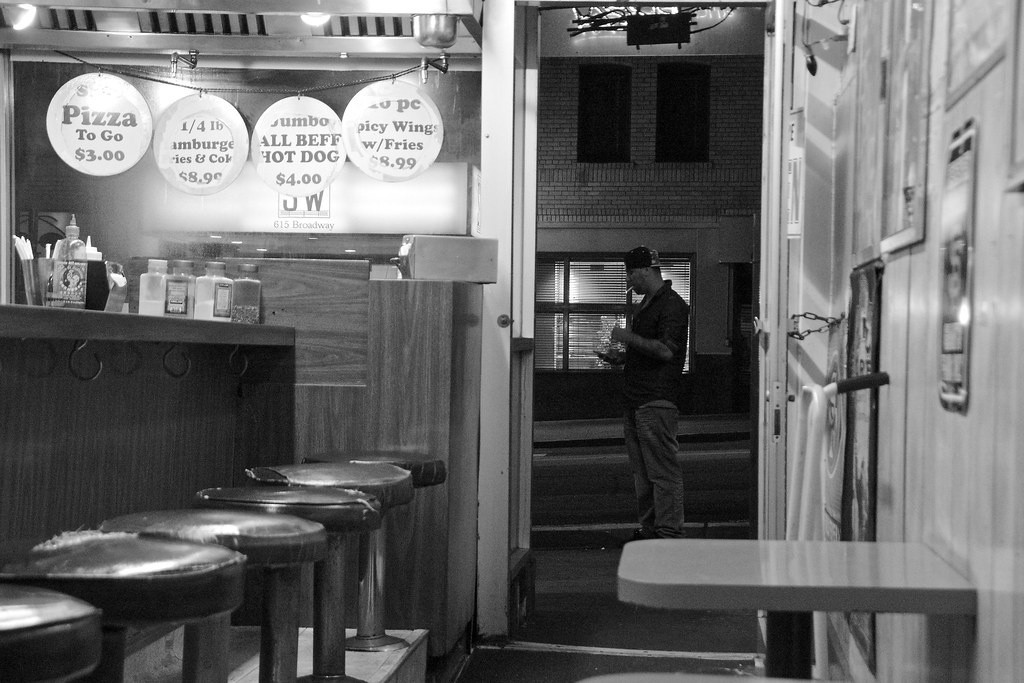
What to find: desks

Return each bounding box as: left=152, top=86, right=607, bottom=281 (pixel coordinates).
left=615, top=538, right=976, bottom=683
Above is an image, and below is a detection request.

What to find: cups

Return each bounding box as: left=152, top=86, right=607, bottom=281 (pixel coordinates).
left=20, top=258, right=50, bottom=306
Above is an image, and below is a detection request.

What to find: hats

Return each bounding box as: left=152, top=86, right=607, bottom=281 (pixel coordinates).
left=624, top=245, right=666, bottom=270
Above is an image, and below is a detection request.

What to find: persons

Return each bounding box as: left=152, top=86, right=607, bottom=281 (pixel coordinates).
left=597, top=245, right=690, bottom=549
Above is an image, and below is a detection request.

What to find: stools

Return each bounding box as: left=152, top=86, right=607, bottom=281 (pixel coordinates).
left=100, top=507, right=327, bottom=683
left=0, top=584, right=105, bottom=683
left=194, top=486, right=381, bottom=683
left=301, top=447, right=447, bottom=654
left=0, top=527, right=248, bottom=683
left=243, top=463, right=414, bottom=683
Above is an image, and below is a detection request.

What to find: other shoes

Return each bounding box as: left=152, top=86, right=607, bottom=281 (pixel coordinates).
left=618, top=528, right=664, bottom=549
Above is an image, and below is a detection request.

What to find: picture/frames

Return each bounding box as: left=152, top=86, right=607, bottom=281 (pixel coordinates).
left=837, top=261, right=883, bottom=679
left=1000, top=0, right=1024, bottom=193
left=943, top=0, right=1007, bottom=113
left=877, top=0, right=935, bottom=255
left=849, top=0, right=885, bottom=268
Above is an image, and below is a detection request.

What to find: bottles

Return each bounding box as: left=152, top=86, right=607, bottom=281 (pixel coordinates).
left=46, top=211, right=128, bottom=312
left=138, top=258, right=266, bottom=325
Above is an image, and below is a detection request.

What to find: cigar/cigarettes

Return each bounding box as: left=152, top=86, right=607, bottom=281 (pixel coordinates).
left=626, top=286, right=634, bottom=294
